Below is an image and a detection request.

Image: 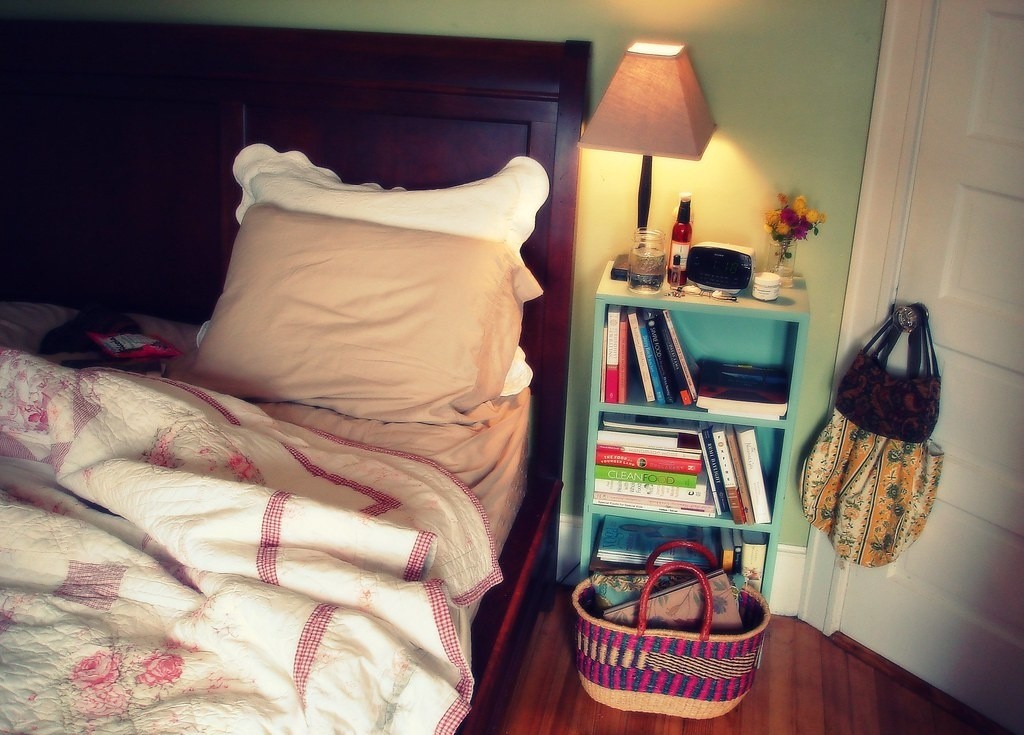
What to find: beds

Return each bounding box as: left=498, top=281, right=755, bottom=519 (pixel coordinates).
left=0, top=15, right=592, bottom=735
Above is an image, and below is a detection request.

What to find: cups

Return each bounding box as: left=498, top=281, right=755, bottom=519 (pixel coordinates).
left=627, top=227, right=667, bottom=295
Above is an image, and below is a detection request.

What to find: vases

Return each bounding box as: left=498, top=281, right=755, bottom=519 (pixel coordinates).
left=766, top=238, right=799, bottom=289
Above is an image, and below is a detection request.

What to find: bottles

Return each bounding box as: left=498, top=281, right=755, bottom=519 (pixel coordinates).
left=671, top=254, right=680, bottom=290
left=667, top=192, right=693, bottom=286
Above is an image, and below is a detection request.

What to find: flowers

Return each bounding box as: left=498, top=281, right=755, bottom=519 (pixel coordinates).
left=764, top=192, right=825, bottom=242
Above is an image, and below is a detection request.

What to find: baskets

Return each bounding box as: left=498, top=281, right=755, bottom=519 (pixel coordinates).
left=571, top=541, right=771, bottom=720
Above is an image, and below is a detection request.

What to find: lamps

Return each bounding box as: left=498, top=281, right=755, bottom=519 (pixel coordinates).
left=577, top=43, right=719, bottom=286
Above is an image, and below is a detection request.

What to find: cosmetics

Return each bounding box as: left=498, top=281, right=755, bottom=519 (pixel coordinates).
left=752, top=271, right=782, bottom=301
left=670, top=254, right=681, bottom=290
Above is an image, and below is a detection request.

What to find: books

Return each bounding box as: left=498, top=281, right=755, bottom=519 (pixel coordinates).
left=588, top=515, right=769, bottom=593
left=696, top=358, right=787, bottom=419
left=726, top=424, right=756, bottom=524
left=735, top=426, right=771, bottom=524
left=600, top=308, right=627, bottom=404
left=593, top=412, right=731, bottom=519
left=628, top=308, right=697, bottom=405
left=713, top=423, right=747, bottom=525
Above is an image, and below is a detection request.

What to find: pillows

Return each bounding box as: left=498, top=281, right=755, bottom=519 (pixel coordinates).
left=161, top=204, right=544, bottom=425
left=195, top=143, right=549, bottom=396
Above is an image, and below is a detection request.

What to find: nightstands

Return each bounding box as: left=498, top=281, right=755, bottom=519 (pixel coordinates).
left=580, top=260, right=810, bottom=606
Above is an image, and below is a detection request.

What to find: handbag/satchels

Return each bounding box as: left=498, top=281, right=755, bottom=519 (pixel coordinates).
left=835, top=303, right=941, bottom=445
left=799, top=303, right=946, bottom=569
